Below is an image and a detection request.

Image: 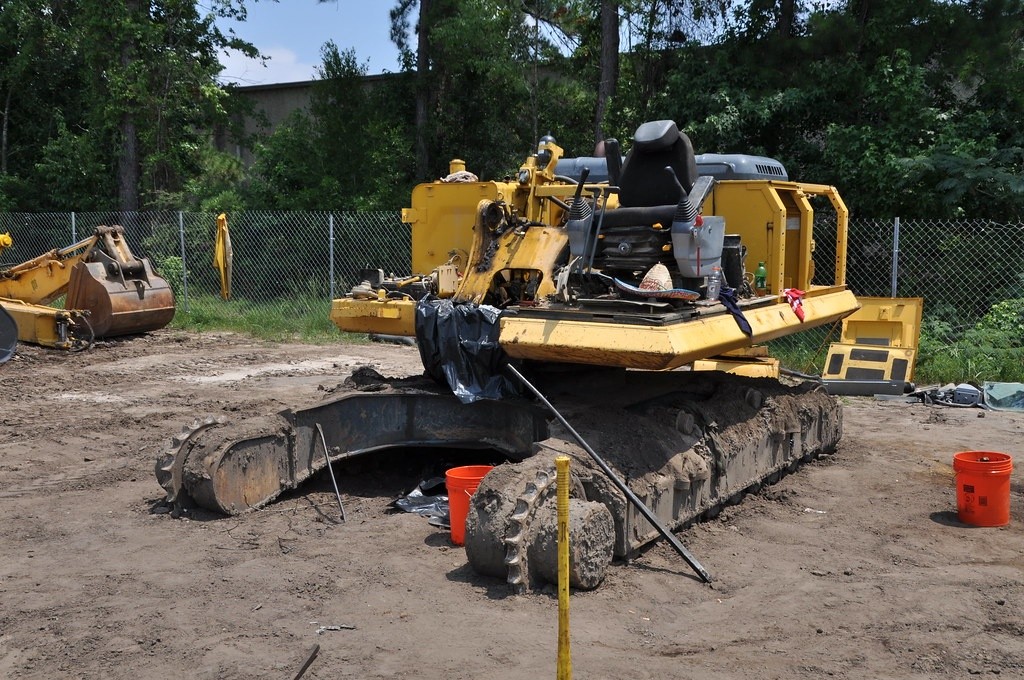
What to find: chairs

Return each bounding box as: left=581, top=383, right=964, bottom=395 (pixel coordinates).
left=593, top=120, right=714, bottom=226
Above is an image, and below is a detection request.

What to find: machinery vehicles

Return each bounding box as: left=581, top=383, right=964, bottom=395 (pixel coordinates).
left=153, top=119, right=862, bottom=599
left=0, top=222, right=177, bottom=370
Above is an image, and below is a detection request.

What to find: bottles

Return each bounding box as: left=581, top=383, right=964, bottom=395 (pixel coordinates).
left=706, top=266, right=721, bottom=301
left=755, top=262, right=767, bottom=297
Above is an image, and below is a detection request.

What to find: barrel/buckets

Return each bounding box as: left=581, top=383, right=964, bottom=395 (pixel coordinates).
left=952, top=450, right=1013, bottom=528
left=445, top=465, right=496, bottom=546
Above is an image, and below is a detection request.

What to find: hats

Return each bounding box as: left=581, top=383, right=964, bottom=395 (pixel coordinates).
left=614, top=262, right=700, bottom=300
